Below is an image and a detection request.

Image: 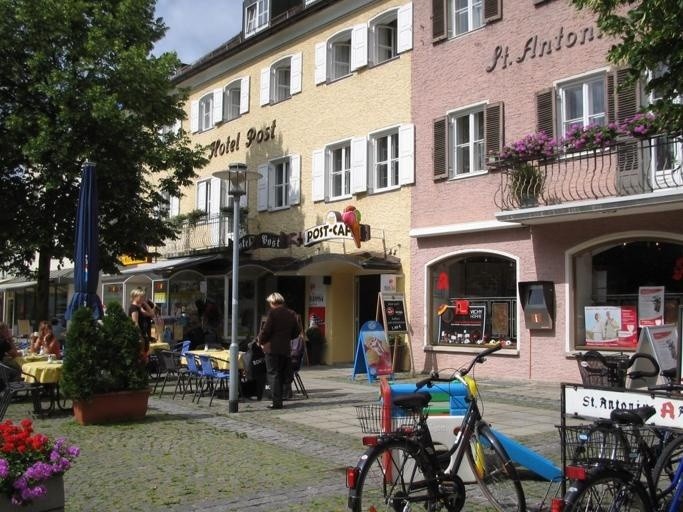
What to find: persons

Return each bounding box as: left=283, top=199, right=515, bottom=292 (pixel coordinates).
left=127, top=286, right=158, bottom=361
left=199, top=294, right=224, bottom=347
left=289, top=332, right=304, bottom=401
left=257, top=291, right=303, bottom=411
left=0, top=323, right=23, bottom=392
left=30, top=320, right=61, bottom=414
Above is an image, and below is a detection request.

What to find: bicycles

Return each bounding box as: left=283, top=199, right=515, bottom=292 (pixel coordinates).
left=551, top=407, right=683, bottom=511
left=555, top=385, right=683, bottom=468
left=542, top=351, right=659, bottom=483
left=346, top=340, right=526, bottom=512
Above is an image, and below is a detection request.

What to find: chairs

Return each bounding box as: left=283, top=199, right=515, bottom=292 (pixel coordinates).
left=181, top=352, right=215, bottom=402
left=196, top=355, right=244, bottom=406
left=159, top=349, right=192, bottom=399
left=171, top=341, right=192, bottom=376
left=296, top=349, right=308, bottom=398
left=0, top=363, right=43, bottom=420
left=0, top=364, right=39, bottom=414
left=148, top=362, right=164, bottom=394
left=291, top=378, right=300, bottom=395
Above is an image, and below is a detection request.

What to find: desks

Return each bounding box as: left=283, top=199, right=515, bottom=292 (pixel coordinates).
left=150, top=343, right=169, bottom=352
left=21, top=362, right=62, bottom=383
left=181, top=350, right=246, bottom=371
left=7, top=356, right=48, bottom=361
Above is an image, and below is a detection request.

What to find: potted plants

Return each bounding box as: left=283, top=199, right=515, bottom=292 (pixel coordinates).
left=506, top=162, right=548, bottom=209
left=59, top=301, right=149, bottom=425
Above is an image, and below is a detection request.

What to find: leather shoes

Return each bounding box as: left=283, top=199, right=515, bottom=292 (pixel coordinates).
left=267, top=403, right=284, bottom=409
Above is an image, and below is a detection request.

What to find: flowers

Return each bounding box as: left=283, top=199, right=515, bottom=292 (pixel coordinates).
left=620, top=115, right=660, bottom=138
left=492, top=133, right=556, bottom=159
left=559, top=123, right=616, bottom=153
left=0, top=417, right=80, bottom=507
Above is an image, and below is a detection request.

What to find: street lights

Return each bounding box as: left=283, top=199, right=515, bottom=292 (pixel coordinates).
left=211, top=163, right=264, bottom=414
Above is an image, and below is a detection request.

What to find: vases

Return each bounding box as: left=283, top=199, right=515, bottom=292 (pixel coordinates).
left=1, top=475, right=65, bottom=512
